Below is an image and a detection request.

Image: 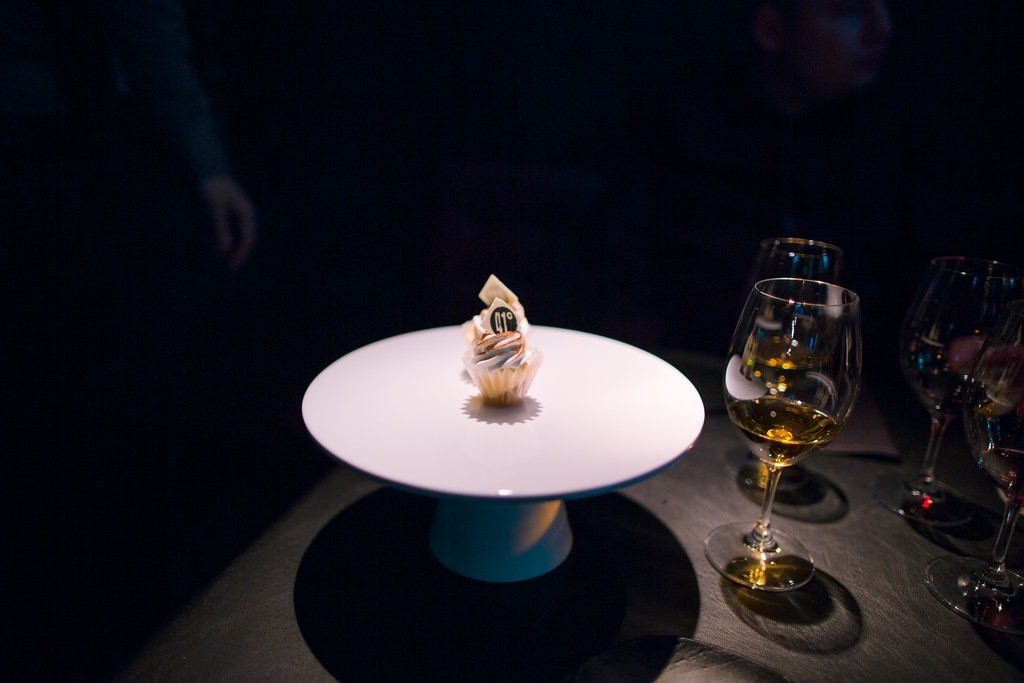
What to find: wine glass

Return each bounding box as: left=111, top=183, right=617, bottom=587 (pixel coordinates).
left=923, top=299, right=1024, bottom=635
left=704, top=238, right=861, bottom=591
left=873, top=257, right=1024, bottom=522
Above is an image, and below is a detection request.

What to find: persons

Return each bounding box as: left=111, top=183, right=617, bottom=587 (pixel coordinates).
left=615, top=0, right=1024, bottom=424
left=0, top=0, right=259, bottom=638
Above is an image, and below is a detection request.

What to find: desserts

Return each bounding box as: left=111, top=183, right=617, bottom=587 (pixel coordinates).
left=459, top=274, right=544, bottom=408
